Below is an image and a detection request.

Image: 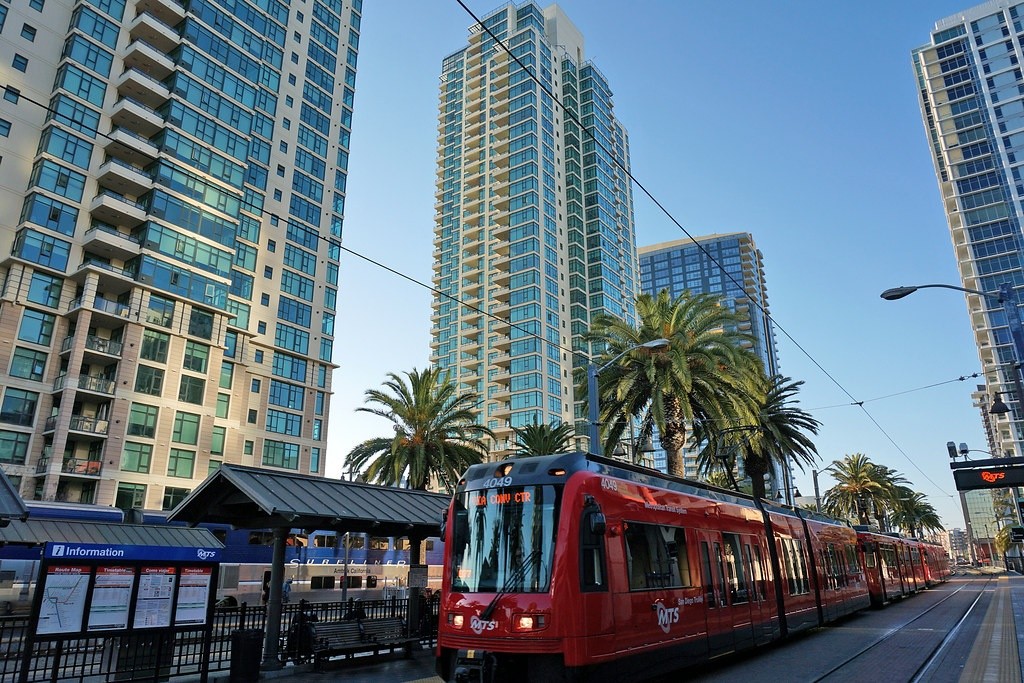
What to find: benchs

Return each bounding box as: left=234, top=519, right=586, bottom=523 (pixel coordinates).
left=0, top=600, right=31, bottom=621
left=307, top=618, right=380, bottom=674
left=355, top=615, right=420, bottom=666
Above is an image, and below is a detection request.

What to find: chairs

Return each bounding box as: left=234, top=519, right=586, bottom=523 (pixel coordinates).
left=490, top=542, right=685, bottom=587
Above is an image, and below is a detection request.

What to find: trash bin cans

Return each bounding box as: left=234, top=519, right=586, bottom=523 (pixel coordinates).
left=230, top=628, right=265, bottom=683
left=97, top=633, right=174, bottom=683
left=367, top=575, right=377, bottom=588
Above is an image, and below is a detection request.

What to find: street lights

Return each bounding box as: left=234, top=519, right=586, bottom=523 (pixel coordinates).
left=989, top=389, right=1024, bottom=414
left=968, top=522, right=996, bottom=569
left=975, top=511, right=1009, bottom=571
left=880, top=284, right=1024, bottom=378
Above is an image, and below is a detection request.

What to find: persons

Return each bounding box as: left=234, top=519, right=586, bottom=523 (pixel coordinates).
left=344, top=598, right=366, bottom=619
left=419, top=589, right=441, bottom=632
left=292, top=601, right=318, bottom=623
left=283, top=579, right=292, bottom=603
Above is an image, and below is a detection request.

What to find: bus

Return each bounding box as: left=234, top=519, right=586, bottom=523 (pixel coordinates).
left=435, top=450, right=951, bottom=683
